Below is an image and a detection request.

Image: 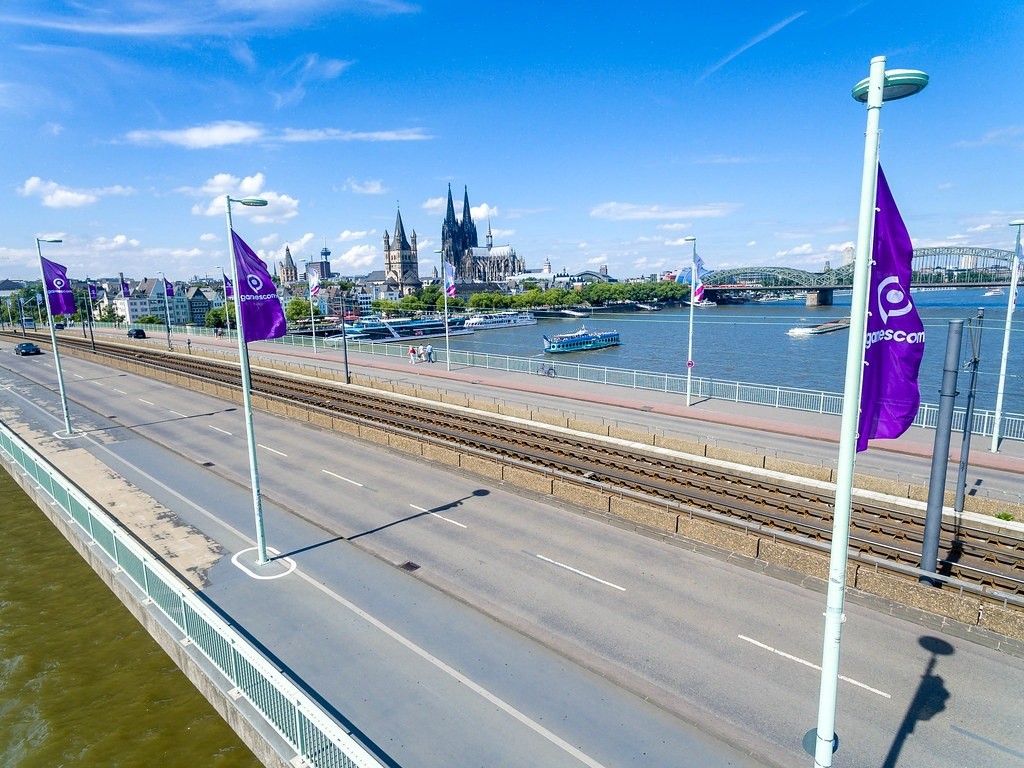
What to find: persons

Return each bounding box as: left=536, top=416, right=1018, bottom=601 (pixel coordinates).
left=408, top=343, right=434, bottom=364
left=214, top=327, right=223, bottom=340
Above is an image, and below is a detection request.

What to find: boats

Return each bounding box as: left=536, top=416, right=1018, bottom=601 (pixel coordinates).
left=463, top=312, right=537, bottom=330
left=324, top=315, right=474, bottom=346
left=778, top=292, right=807, bottom=301
left=756, top=294, right=779, bottom=302
left=984, top=289, right=1005, bottom=297
left=799, top=316, right=850, bottom=332
left=543, top=324, right=621, bottom=353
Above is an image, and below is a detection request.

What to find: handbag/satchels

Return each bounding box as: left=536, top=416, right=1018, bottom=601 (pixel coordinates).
left=413, top=350, right=416, bottom=354
left=418, top=354, right=420, bottom=358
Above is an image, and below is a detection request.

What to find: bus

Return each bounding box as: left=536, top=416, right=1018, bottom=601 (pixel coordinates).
left=17, top=317, right=36, bottom=329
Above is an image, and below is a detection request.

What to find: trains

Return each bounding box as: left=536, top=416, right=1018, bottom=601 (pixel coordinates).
left=704, top=284, right=763, bottom=287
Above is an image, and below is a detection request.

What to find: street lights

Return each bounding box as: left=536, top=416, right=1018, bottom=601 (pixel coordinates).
left=214, top=266, right=231, bottom=341
left=156, top=270, right=172, bottom=337
left=684, top=236, right=698, bottom=405
left=151, top=302, right=171, bottom=351
left=31, top=289, right=42, bottom=327
left=992, top=218, right=1024, bottom=452
left=311, top=295, right=350, bottom=384
left=35, top=238, right=72, bottom=433
left=3, top=299, right=13, bottom=328
left=86, top=282, right=95, bottom=327
left=433, top=250, right=450, bottom=373
left=811, top=67, right=927, bottom=768
left=299, top=258, right=316, bottom=354
left=226, top=198, right=268, bottom=563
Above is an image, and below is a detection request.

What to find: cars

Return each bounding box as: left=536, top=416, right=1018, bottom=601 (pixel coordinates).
left=54, top=324, right=65, bottom=330
left=127, top=329, right=146, bottom=339
left=14, top=343, right=41, bottom=357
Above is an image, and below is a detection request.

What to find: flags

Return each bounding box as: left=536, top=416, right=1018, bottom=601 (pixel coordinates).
left=165, top=280, right=174, bottom=296
left=692, top=253, right=706, bottom=302
left=309, top=268, right=319, bottom=295
left=41, top=257, right=78, bottom=314
left=225, top=276, right=234, bottom=295
left=6, top=294, right=43, bottom=306
left=446, top=262, right=456, bottom=298
left=231, top=230, right=286, bottom=341
left=858, top=165, right=925, bottom=453
left=90, top=285, right=98, bottom=298
left=123, top=283, right=131, bottom=297
